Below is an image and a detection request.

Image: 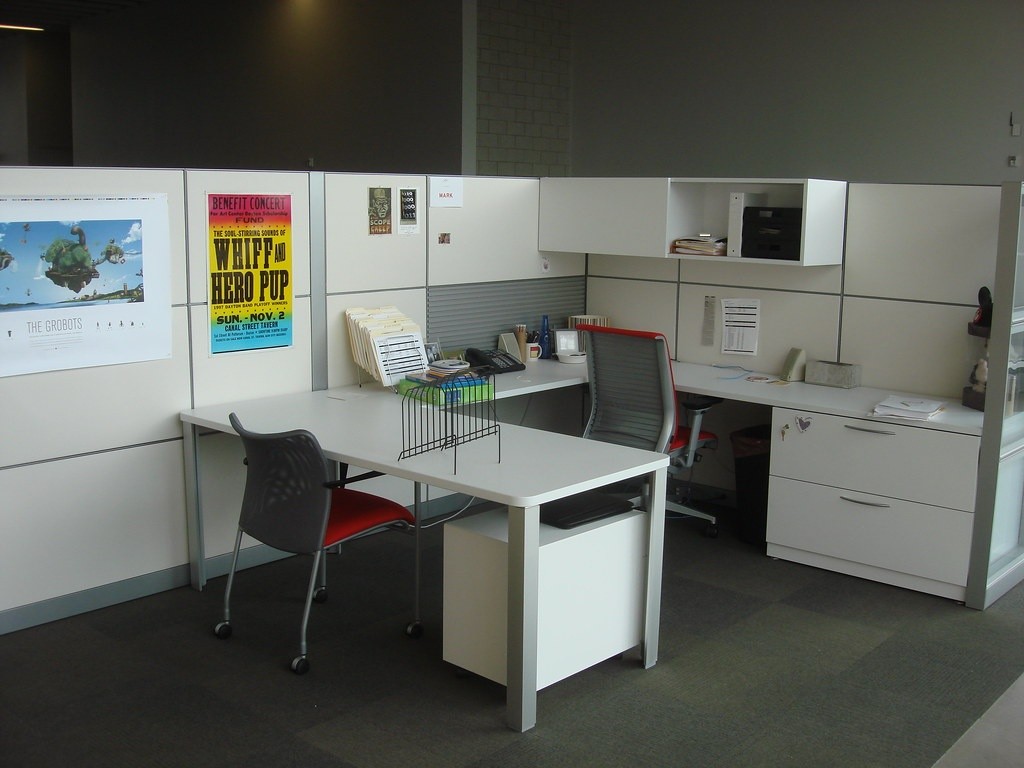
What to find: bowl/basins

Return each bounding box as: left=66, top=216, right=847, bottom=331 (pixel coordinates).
left=556, top=352, right=586, bottom=363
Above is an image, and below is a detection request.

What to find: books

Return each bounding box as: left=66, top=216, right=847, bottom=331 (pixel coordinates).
left=676, top=235, right=727, bottom=256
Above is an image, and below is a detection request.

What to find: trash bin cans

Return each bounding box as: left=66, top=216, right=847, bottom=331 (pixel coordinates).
left=730, top=423, right=772, bottom=544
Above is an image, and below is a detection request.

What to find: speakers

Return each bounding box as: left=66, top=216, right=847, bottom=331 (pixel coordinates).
left=779, top=348, right=806, bottom=381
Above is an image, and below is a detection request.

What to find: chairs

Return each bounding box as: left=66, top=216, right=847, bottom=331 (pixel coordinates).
left=573, top=324, right=729, bottom=541
left=212, top=414, right=423, bottom=677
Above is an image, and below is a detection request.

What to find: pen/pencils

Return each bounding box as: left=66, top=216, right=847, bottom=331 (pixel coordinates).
left=526, top=330, right=541, bottom=343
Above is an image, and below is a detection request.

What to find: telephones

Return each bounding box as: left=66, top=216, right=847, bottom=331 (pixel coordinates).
left=465, top=347, right=526, bottom=374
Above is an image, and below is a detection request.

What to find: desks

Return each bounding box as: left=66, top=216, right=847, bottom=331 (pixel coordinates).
left=335, top=344, right=1024, bottom=613
left=179, top=389, right=672, bottom=731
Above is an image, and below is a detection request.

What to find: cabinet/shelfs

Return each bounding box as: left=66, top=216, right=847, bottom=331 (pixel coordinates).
left=536, top=177, right=849, bottom=268
left=766, top=406, right=1024, bottom=604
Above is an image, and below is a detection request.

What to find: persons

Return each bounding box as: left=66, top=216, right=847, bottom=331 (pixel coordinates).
left=427, top=345, right=440, bottom=363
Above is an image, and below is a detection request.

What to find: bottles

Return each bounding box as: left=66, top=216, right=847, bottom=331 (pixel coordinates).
left=538, top=315, right=551, bottom=359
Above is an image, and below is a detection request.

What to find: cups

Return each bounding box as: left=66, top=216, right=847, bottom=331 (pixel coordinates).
left=526, top=343, right=542, bottom=362
